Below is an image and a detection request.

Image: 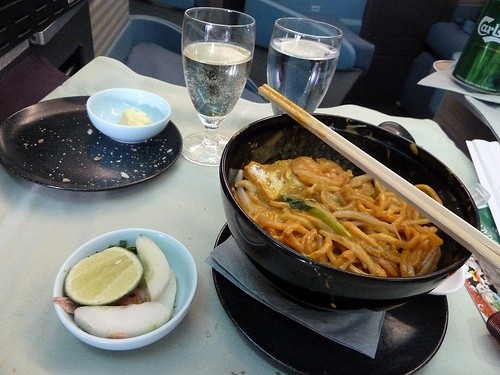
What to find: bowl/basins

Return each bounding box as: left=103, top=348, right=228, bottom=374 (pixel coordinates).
left=52, top=228, right=198, bottom=351
left=86, top=88, right=172, bottom=144
left=218, top=112, right=482, bottom=314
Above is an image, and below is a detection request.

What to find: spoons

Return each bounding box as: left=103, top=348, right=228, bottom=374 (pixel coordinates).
left=377, top=120, right=416, bottom=146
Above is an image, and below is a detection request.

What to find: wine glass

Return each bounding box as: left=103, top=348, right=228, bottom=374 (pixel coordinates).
left=181, top=7, right=257, bottom=167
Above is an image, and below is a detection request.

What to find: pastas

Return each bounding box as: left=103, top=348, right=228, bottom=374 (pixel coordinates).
left=230, top=157, right=444, bottom=278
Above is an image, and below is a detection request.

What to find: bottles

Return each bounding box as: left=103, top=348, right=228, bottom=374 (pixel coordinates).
left=450, top=0, right=500, bottom=97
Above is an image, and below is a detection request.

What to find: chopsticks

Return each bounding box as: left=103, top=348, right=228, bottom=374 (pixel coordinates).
left=258, top=84, right=500, bottom=271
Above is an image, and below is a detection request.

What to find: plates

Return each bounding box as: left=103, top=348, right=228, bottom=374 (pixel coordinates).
left=210, top=222, right=449, bottom=374
left=1, top=95, right=183, bottom=192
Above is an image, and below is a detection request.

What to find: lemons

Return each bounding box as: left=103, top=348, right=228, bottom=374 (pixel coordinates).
left=63, top=246, right=143, bottom=305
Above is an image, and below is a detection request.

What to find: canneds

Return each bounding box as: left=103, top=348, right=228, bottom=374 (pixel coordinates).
left=450, top=0, right=500, bottom=97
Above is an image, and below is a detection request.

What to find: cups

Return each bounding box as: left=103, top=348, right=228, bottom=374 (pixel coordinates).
left=266, top=17, right=344, bottom=116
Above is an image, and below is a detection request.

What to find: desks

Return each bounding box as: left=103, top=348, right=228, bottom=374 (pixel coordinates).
left=0, top=0, right=500, bottom=375
